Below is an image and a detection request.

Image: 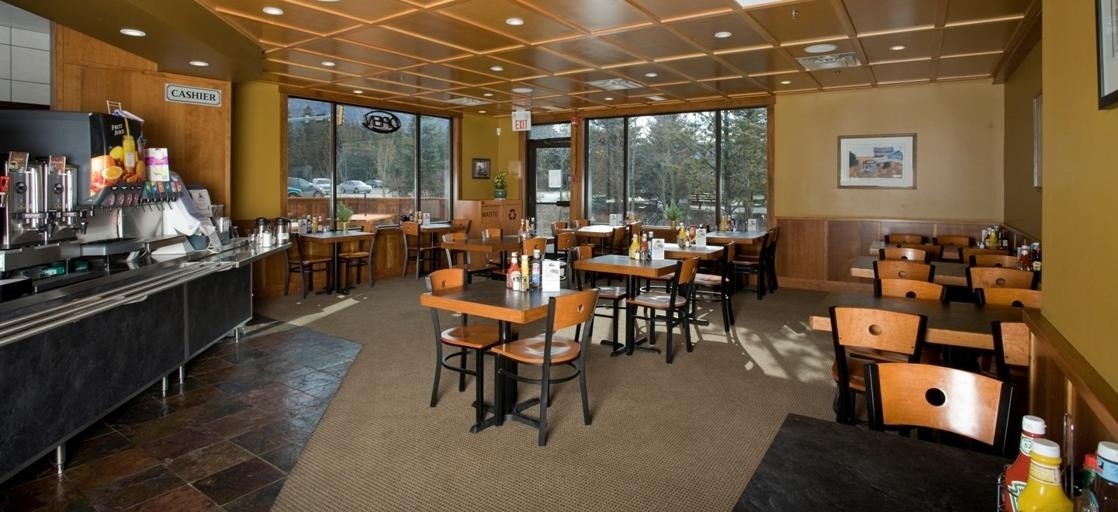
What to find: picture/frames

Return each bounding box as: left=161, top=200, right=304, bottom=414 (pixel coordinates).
left=837, top=133, right=917, bottom=190
left=1032, top=90, right=1042, bottom=192
left=472, top=158, right=490, bottom=179
left=1094, top=0, right=1118, bottom=109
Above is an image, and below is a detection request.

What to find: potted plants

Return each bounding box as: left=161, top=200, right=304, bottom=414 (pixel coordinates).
left=493, top=170, right=507, bottom=200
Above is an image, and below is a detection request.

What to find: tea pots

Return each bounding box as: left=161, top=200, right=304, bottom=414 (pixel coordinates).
left=252, top=216, right=292, bottom=248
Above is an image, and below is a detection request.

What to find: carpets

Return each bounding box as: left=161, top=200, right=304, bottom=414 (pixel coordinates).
left=257, top=274, right=827, bottom=512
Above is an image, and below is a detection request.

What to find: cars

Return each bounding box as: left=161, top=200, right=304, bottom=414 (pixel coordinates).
left=287, top=177, right=385, bottom=198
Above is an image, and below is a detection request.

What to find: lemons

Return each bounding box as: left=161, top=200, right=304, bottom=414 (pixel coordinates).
left=102, top=166, right=123, bottom=180
left=110, top=146, right=123, bottom=166
left=136, top=160, right=145, bottom=178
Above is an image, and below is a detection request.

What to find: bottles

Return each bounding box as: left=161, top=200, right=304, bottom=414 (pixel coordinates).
left=623, top=211, right=637, bottom=225
left=1002, top=413, right=1118, bottom=512
left=516, top=217, right=537, bottom=244
left=718, top=214, right=737, bottom=234
left=677, top=221, right=696, bottom=250
left=296, top=214, right=332, bottom=235
left=409, top=208, right=424, bottom=226
left=505, top=249, right=543, bottom=292
left=981, top=223, right=1042, bottom=276
left=628, top=230, right=655, bottom=261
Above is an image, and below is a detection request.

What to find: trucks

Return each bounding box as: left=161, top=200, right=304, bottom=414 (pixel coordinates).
left=870, top=147, right=894, bottom=161
